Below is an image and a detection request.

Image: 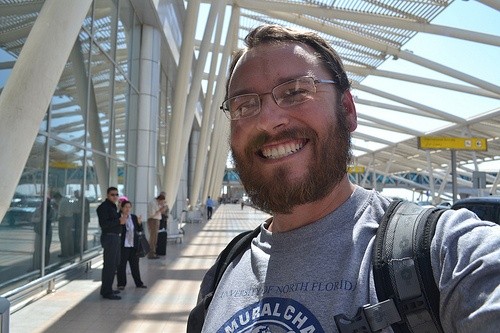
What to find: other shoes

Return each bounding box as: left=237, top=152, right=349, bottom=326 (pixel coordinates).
left=112, top=290, right=120, bottom=294
left=118, top=285, right=123, bottom=289
left=149, top=256, right=160, bottom=259
left=102, top=295, right=121, bottom=300
left=136, top=285, right=147, bottom=288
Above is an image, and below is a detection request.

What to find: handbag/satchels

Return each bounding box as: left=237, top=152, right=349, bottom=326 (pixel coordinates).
left=137, top=234, right=150, bottom=257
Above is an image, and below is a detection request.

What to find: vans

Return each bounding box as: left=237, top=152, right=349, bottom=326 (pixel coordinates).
left=450, top=195, right=500, bottom=224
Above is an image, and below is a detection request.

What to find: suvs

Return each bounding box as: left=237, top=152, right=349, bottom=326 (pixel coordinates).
left=5, top=183, right=59, bottom=227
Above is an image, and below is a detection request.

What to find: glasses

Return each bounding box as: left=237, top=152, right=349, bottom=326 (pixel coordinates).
left=220, top=76, right=338, bottom=121
left=109, top=194, right=118, bottom=196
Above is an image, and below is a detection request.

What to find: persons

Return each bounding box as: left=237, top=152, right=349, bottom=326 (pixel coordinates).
left=183, top=24, right=500, bottom=333
left=146, top=190, right=170, bottom=260
left=205, top=195, right=214, bottom=220
left=95, top=186, right=148, bottom=301
left=239, top=198, right=245, bottom=210
left=31, top=189, right=91, bottom=271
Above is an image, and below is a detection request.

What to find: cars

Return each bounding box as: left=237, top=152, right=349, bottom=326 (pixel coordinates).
left=436, top=200, right=453, bottom=210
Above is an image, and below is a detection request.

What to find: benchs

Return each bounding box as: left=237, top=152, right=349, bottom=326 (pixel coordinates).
left=187, top=211, right=204, bottom=225
left=168, top=217, right=184, bottom=245
